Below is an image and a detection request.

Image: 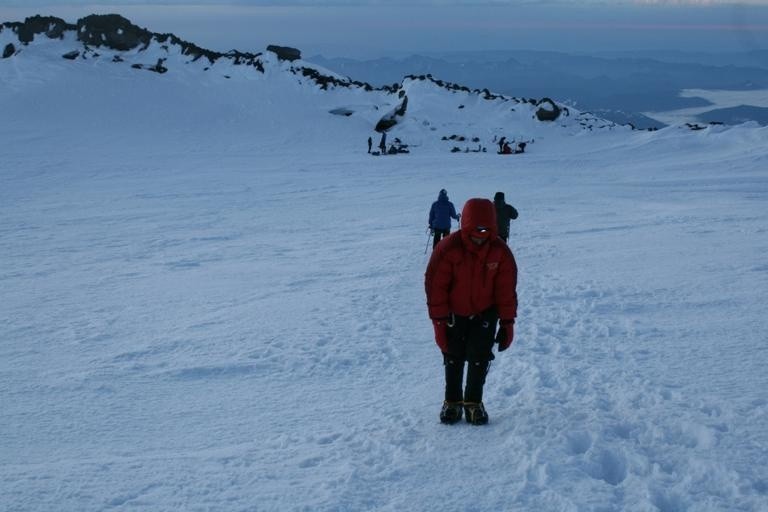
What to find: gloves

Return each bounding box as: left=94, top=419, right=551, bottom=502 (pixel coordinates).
left=495, top=320, right=514, bottom=351
left=433, top=319, right=447, bottom=353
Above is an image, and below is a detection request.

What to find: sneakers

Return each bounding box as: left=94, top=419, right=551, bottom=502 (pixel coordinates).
left=440, top=400, right=463, bottom=424
left=464, top=400, right=488, bottom=425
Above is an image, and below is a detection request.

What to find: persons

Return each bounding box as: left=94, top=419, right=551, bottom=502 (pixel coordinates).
left=427, top=188, right=461, bottom=250
left=424, top=197, right=519, bottom=426
left=492, top=192, right=519, bottom=245
left=379, top=130, right=386, bottom=155
left=498, top=137, right=526, bottom=155
left=367, top=137, right=372, bottom=154
